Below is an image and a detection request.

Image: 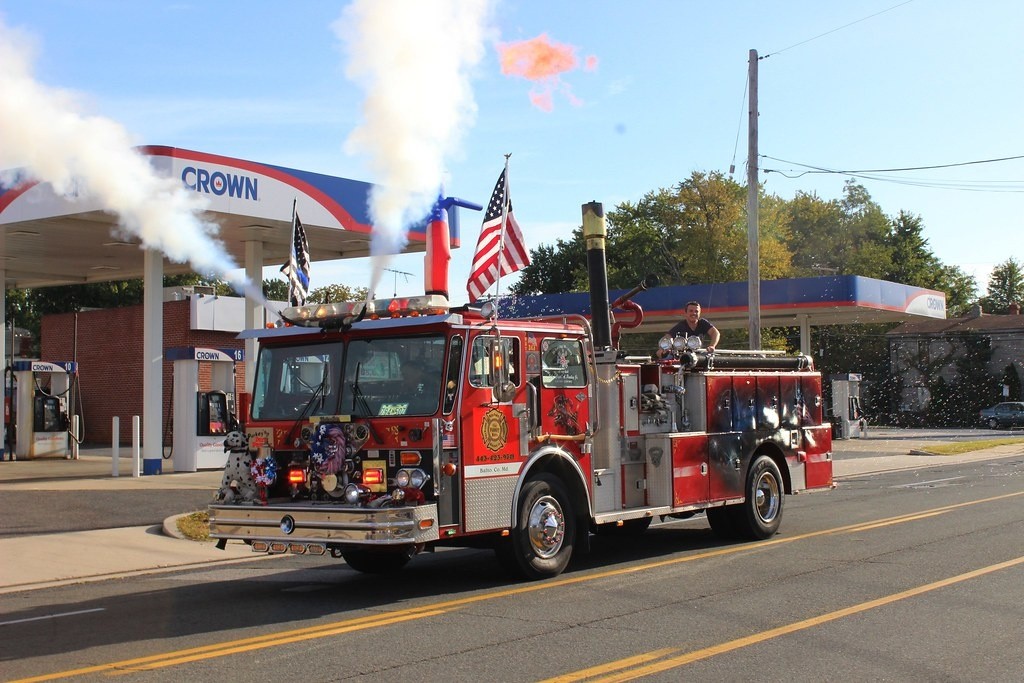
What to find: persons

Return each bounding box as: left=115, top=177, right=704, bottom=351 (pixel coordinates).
left=656, top=300, right=721, bottom=364
left=390, top=360, right=440, bottom=415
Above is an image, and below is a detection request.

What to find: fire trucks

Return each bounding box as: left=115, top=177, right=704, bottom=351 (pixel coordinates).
left=206, top=200, right=836, bottom=581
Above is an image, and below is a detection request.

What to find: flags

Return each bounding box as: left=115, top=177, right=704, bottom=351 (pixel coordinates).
left=467, top=168, right=530, bottom=304
left=290, top=215, right=310, bottom=305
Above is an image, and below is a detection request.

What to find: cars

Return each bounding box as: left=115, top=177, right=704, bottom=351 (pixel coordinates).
left=977, top=400, right=1024, bottom=430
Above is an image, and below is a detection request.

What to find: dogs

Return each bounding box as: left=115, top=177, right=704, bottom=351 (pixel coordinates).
left=213, top=431, right=258, bottom=502
